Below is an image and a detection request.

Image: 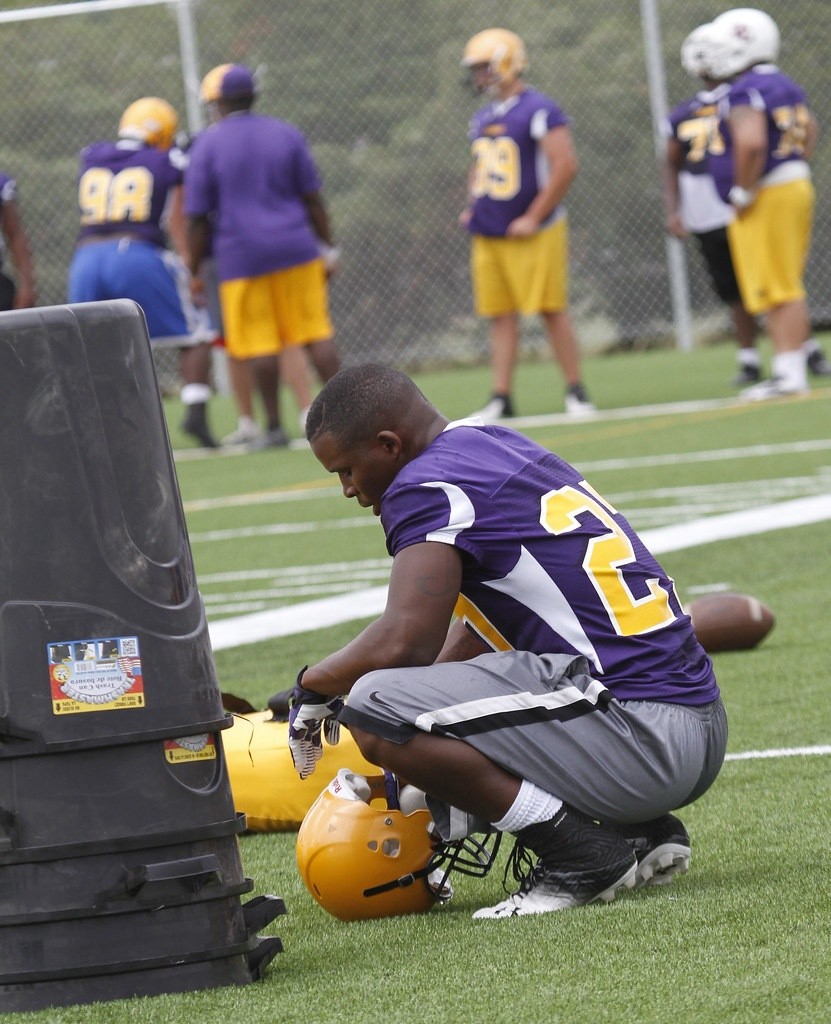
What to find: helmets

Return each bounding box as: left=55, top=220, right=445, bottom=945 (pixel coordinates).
left=680, top=22, right=715, bottom=77
left=461, top=27, right=527, bottom=88
left=295, top=774, right=503, bottom=922
left=118, top=96, right=179, bottom=153
left=706, top=8, right=781, bottom=80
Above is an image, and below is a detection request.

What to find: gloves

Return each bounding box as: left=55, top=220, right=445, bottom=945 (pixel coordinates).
left=289, top=665, right=345, bottom=780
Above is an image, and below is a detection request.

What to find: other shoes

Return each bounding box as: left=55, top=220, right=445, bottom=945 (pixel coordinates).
left=563, top=390, right=611, bottom=413
left=184, top=415, right=219, bottom=450
left=474, top=398, right=510, bottom=423
left=742, top=369, right=805, bottom=403
left=734, top=361, right=758, bottom=390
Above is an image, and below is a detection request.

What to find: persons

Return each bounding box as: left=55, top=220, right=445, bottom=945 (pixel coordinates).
left=269, top=359, right=728, bottom=919
left=661, top=8, right=830, bottom=405
left=0, top=170, right=37, bottom=311
left=459, top=26, right=598, bottom=420
left=163, top=61, right=345, bottom=453
left=63, top=94, right=216, bottom=449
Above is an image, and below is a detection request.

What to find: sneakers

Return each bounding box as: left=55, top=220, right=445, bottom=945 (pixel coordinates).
left=606, top=809, right=691, bottom=890
left=472, top=800, right=636, bottom=918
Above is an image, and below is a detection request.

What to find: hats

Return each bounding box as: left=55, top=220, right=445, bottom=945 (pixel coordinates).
left=199, top=62, right=253, bottom=103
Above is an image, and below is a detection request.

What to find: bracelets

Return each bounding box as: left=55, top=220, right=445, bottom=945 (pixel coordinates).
left=727, top=185, right=756, bottom=208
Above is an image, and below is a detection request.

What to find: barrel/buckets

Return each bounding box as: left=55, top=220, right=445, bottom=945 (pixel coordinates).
left=0, top=296, right=286, bottom=1017
